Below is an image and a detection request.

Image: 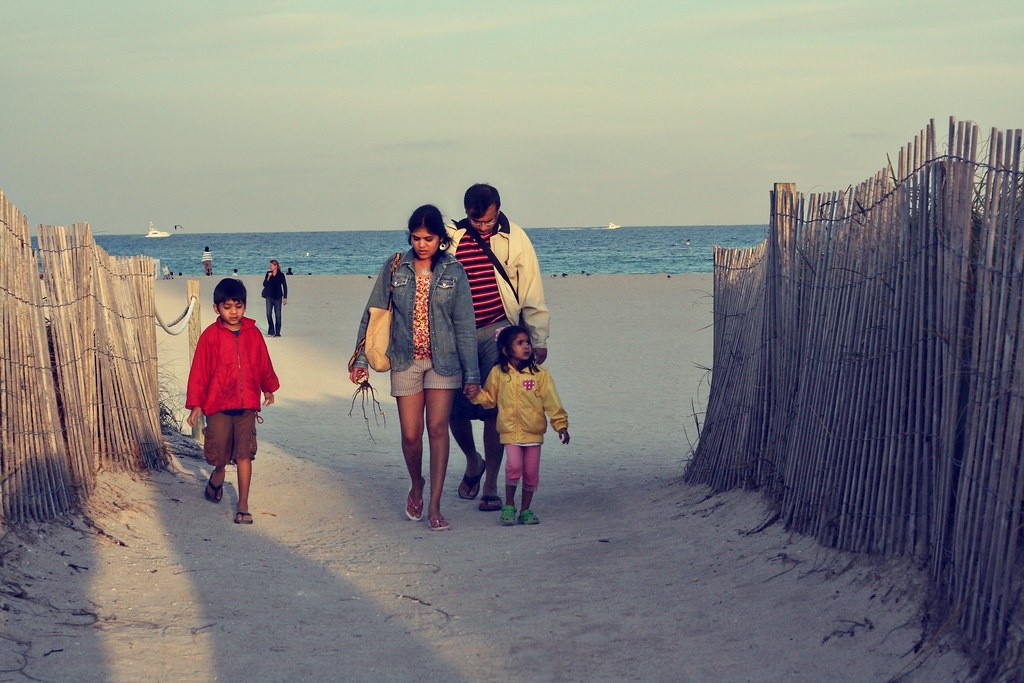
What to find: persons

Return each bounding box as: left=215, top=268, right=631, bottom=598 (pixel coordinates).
left=348, top=204, right=480, bottom=530
left=170, top=246, right=213, bottom=275
left=233, top=269, right=239, bottom=276
left=468, top=325, right=571, bottom=526
left=185, top=277, right=281, bottom=524
left=286, top=268, right=294, bottom=275
left=439, top=183, right=550, bottom=511
left=262, top=260, right=287, bottom=337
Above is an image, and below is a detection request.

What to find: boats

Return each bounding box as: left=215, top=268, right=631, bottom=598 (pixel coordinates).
left=146, top=221, right=172, bottom=238
left=609, top=223, right=622, bottom=229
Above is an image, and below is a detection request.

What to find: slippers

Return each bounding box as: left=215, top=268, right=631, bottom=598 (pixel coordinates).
left=517, top=510, right=539, bottom=525
left=479, top=494, right=503, bottom=512
left=405, top=485, right=423, bottom=521
left=428, top=515, right=451, bottom=531
left=204, top=470, right=223, bottom=503
left=234, top=512, right=253, bottom=524
left=458, top=459, right=486, bottom=499
left=499, top=505, right=517, bottom=524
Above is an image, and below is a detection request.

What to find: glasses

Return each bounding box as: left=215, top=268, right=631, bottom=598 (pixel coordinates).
left=466, top=210, right=498, bottom=226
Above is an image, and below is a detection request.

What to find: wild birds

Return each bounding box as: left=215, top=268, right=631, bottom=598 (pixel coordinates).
left=174, top=224, right=183, bottom=229
left=667, top=275, right=671, bottom=278
left=367, top=276, right=374, bottom=279
left=582, top=270, right=591, bottom=276
left=562, top=273, right=567, bottom=276
left=550, top=274, right=557, bottom=278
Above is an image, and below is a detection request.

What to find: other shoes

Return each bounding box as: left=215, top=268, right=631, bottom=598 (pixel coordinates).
left=267, top=333, right=274, bottom=337
left=274, top=334, right=281, bottom=337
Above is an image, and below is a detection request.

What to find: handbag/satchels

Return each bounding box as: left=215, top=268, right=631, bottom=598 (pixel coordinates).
left=262, top=288, right=266, bottom=298
left=364, top=252, right=401, bottom=373
